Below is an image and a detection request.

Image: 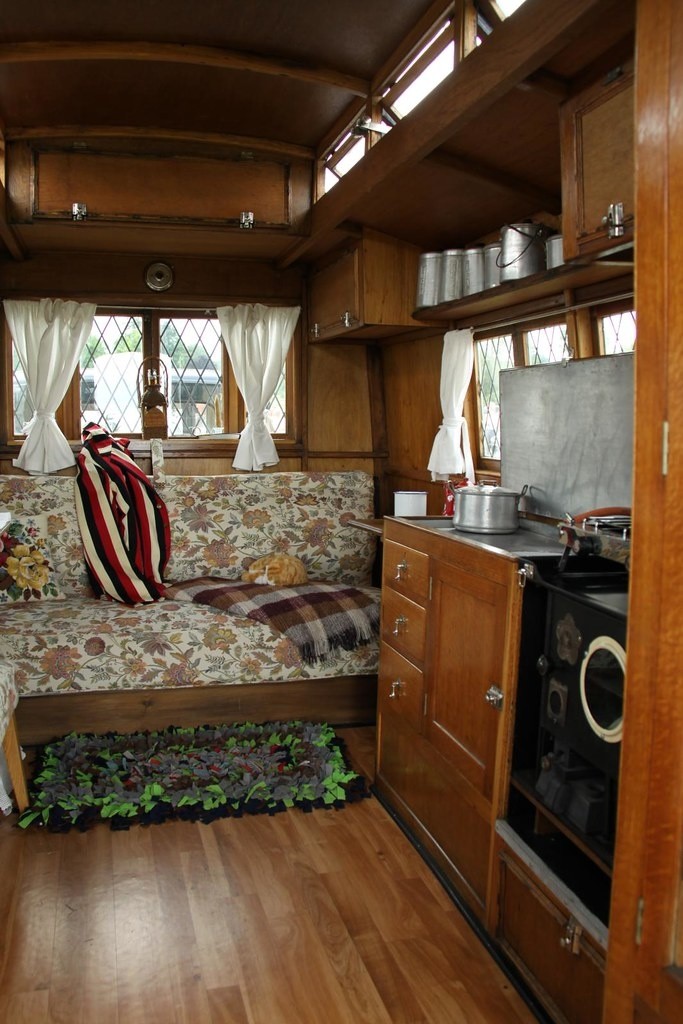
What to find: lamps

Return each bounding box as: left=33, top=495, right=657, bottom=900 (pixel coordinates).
left=136, top=356, right=169, bottom=440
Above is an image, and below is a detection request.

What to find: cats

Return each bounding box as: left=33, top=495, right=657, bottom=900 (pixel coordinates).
left=241, top=555, right=309, bottom=587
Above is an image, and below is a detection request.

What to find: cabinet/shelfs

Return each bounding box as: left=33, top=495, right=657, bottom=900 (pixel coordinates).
left=373, top=508, right=576, bottom=941
left=306, top=225, right=448, bottom=346
left=557, top=58, right=636, bottom=269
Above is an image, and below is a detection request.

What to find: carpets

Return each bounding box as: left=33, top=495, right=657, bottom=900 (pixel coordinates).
left=15, top=720, right=373, bottom=831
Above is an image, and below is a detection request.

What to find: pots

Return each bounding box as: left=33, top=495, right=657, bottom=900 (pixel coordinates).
left=447, top=480, right=529, bottom=533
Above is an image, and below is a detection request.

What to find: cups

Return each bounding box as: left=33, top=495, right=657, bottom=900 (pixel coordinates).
left=417, top=243, right=501, bottom=306
left=393, top=492, right=427, bottom=516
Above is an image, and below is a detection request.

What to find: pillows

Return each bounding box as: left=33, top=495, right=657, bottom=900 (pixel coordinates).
left=0, top=514, right=67, bottom=605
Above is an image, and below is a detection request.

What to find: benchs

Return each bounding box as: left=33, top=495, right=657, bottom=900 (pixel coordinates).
left=0, top=470, right=384, bottom=817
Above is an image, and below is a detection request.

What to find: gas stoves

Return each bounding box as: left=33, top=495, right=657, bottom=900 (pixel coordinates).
left=558, top=513, right=630, bottom=576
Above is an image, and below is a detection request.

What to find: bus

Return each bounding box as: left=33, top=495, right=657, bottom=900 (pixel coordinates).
left=15, top=367, right=273, bottom=434
